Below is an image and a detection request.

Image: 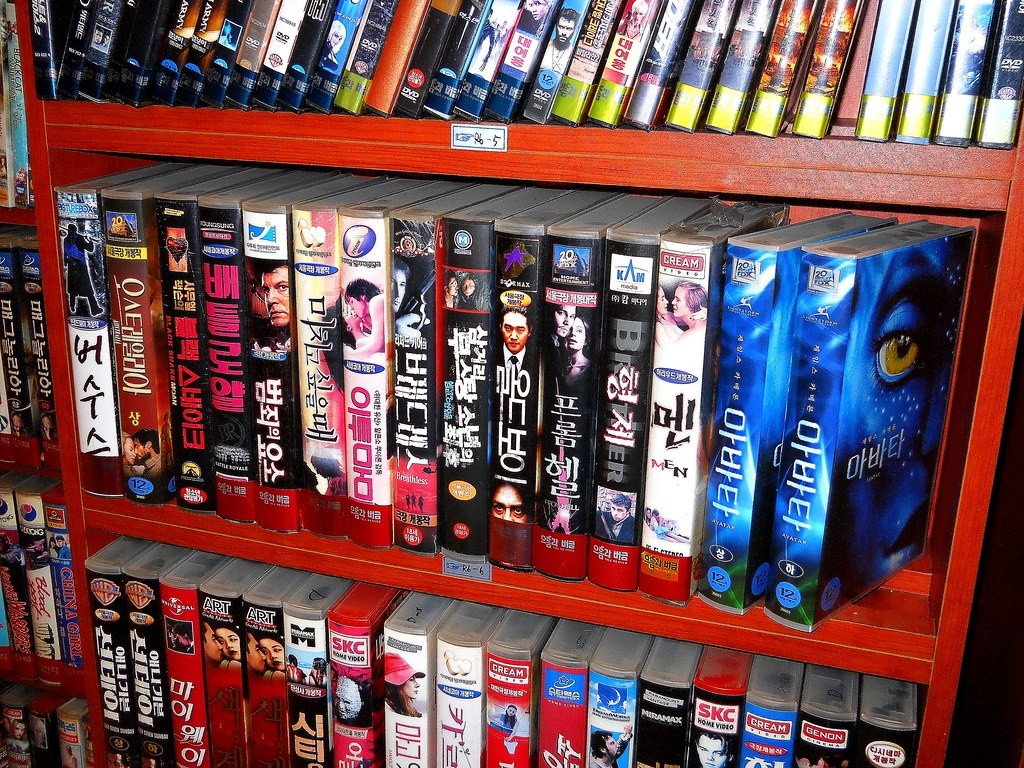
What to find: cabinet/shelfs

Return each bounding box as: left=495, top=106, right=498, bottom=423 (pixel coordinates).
left=0, top=0, right=1024, bottom=768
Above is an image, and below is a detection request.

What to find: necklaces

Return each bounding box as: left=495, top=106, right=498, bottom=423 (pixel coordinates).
left=551, top=43, right=565, bottom=69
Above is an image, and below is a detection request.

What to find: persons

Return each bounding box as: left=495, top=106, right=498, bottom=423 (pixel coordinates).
left=655, top=281, right=707, bottom=347
left=391, top=260, right=430, bottom=346
left=10, top=718, right=26, bottom=739
left=107, top=749, right=131, bottom=768
left=94, top=27, right=111, bottom=46
left=385, top=652, right=426, bottom=717
left=489, top=704, right=519, bottom=742
left=540, top=8, right=580, bottom=73
left=342, top=278, right=385, bottom=357
left=251, top=259, right=291, bottom=353
left=526, top=0, right=553, bottom=37
left=491, top=482, right=531, bottom=523
left=498, top=305, right=534, bottom=370
left=643, top=507, right=691, bottom=544
left=170, top=623, right=194, bottom=653
left=594, top=494, right=634, bottom=542
left=548, top=303, right=590, bottom=378
left=202, top=621, right=328, bottom=686
left=49, top=535, right=71, bottom=559
left=63, top=744, right=78, bottom=768
left=694, top=731, right=733, bottom=768
left=319, top=20, right=347, bottom=71
left=443, top=269, right=478, bottom=308
left=122, top=428, right=162, bottom=478
left=618, top=0, right=648, bottom=40
left=589, top=724, right=633, bottom=768
